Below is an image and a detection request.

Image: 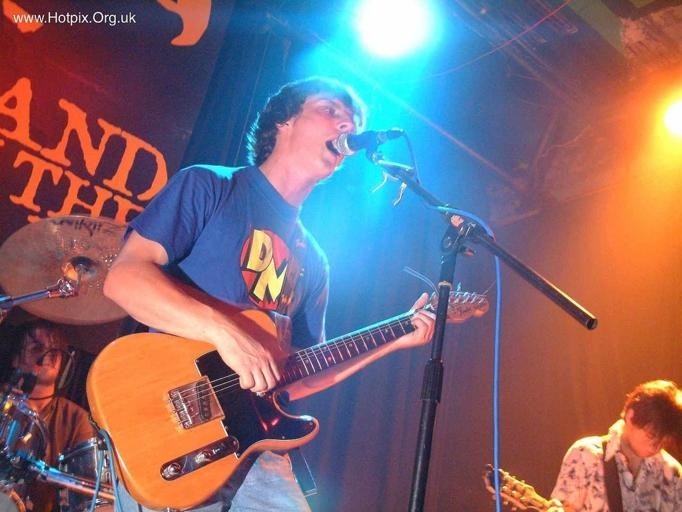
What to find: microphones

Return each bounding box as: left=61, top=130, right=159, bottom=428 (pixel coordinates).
left=335, top=129, right=405, bottom=156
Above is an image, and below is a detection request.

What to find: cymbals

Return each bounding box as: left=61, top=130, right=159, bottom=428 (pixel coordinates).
left=0, top=214, right=130, bottom=327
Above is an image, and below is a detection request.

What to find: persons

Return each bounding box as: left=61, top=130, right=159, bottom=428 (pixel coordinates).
left=102, top=77, right=436, bottom=512
left=0, top=319, right=101, bottom=511
left=551, top=379, right=682, bottom=511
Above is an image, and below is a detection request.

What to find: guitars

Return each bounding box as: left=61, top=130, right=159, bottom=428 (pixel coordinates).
left=85, top=280, right=491, bottom=511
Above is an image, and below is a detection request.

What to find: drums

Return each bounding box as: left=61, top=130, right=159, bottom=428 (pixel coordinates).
left=0, top=394, right=48, bottom=482
left=58, top=437, right=113, bottom=511
left=1, top=481, right=36, bottom=512
